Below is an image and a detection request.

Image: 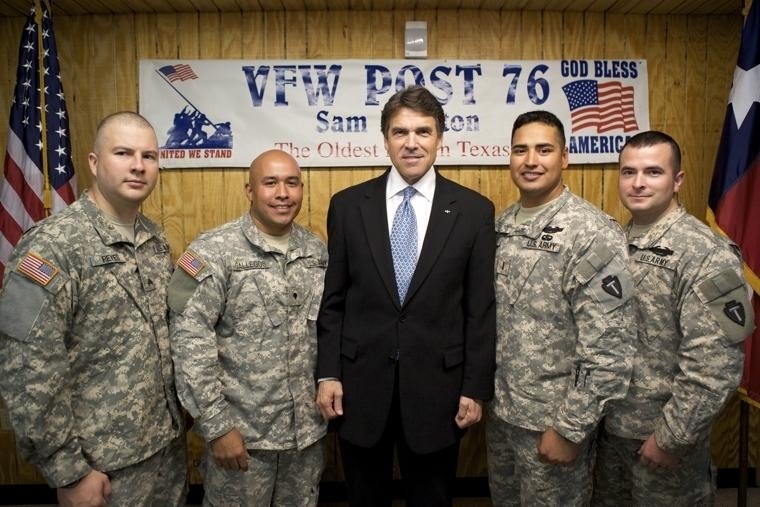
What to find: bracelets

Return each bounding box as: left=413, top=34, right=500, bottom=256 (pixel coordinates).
left=60, top=478, right=81, bottom=489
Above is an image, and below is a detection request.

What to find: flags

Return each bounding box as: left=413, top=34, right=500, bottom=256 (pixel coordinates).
left=563, top=80, right=638, bottom=132
left=707, top=2, right=760, bottom=407
left=160, top=63, right=198, bottom=82
left=0, top=3, right=79, bottom=282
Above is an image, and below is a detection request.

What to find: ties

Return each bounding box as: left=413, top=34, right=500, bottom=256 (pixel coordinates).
left=390, top=187, right=418, bottom=306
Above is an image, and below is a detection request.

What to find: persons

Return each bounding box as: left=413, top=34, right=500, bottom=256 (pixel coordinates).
left=0, top=112, right=193, bottom=505
left=485, top=112, right=638, bottom=506
left=167, top=151, right=330, bottom=507
left=314, top=88, right=496, bottom=506
left=593, top=132, right=759, bottom=505
